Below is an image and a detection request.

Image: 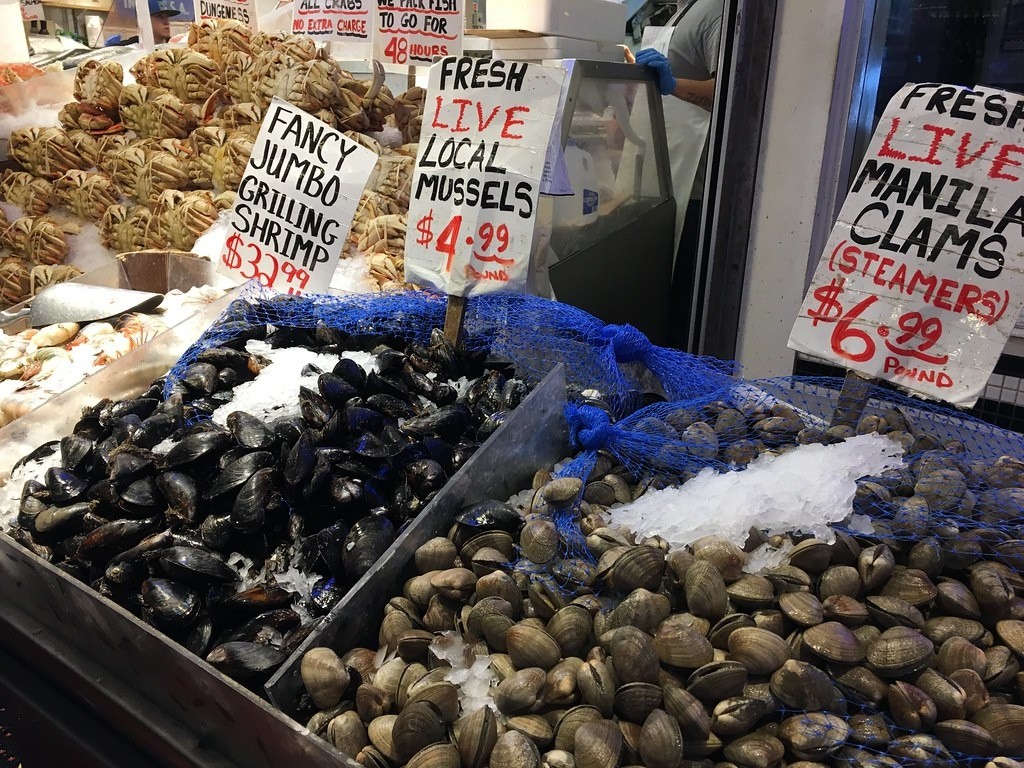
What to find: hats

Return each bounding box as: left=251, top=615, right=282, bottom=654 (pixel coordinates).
left=148, top=0, right=181, bottom=16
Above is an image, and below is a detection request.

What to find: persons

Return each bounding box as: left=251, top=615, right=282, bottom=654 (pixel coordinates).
left=606, top=0, right=723, bottom=347
left=117, top=0, right=181, bottom=46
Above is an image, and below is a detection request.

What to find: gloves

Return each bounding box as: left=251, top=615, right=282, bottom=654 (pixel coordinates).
left=636, top=48, right=676, bottom=95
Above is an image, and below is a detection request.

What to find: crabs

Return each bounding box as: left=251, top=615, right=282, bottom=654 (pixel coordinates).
left=0, top=14, right=436, bottom=316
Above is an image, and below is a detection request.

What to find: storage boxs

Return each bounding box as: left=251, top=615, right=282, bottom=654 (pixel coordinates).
left=485, top=0, right=627, bottom=61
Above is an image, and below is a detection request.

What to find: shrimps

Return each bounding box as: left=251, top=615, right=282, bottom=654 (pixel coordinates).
left=0, top=307, right=177, bottom=430
